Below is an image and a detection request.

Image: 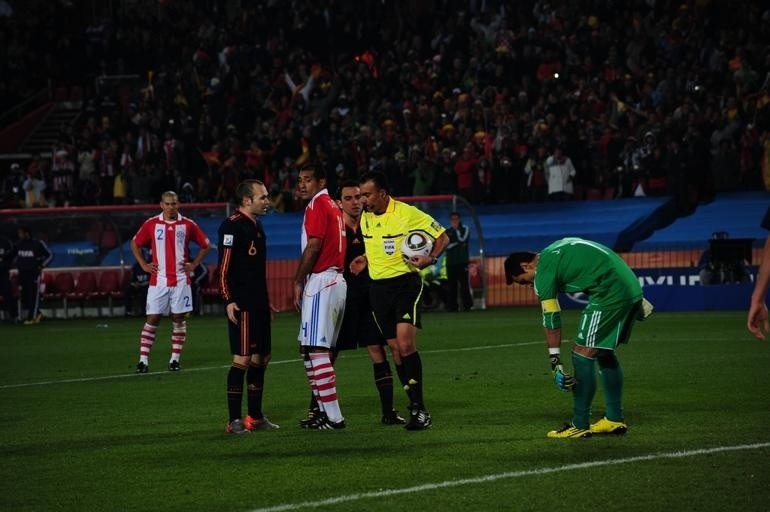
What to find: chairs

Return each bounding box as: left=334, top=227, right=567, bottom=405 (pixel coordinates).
left=11, top=265, right=229, bottom=318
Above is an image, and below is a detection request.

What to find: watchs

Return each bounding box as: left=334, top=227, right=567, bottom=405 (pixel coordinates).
left=428, top=254, right=438, bottom=265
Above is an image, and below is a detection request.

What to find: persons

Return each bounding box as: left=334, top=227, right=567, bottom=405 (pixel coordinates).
left=308, top=178, right=406, bottom=426
left=422, top=254, right=448, bottom=310
left=131, top=191, right=211, bottom=374
left=2, top=2, right=766, bottom=212
left=5, top=226, right=53, bottom=327
left=186, top=245, right=211, bottom=317
left=445, top=212, right=476, bottom=313
left=125, top=252, right=153, bottom=317
left=746, top=205, right=770, bottom=344
left=215, top=178, right=282, bottom=434
left=345, top=168, right=453, bottom=432
left=503, top=236, right=645, bottom=439
left=288, top=160, right=351, bottom=431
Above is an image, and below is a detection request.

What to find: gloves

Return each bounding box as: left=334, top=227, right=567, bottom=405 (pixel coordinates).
left=636, top=298, right=655, bottom=321
left=550, top=355, right=576, bottom=393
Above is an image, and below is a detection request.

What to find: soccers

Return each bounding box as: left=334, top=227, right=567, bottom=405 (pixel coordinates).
left=401, top=231, right=433, bottom=262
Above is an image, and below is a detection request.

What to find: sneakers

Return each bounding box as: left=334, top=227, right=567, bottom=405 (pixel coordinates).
left=225, top=415, right=279, bottom=434
left=300, top=408, right=346, bottom=430
left=136, top=362, right=148, bottom=373
left=590, top=417, right=627, bottom=434
left=405, top=410, right=432, bottom=431
left=547, top=422, right=592, bottom=439
left=382, top=408, right=406, bottom=424
left=169, top=360, right=180, bottom=371
